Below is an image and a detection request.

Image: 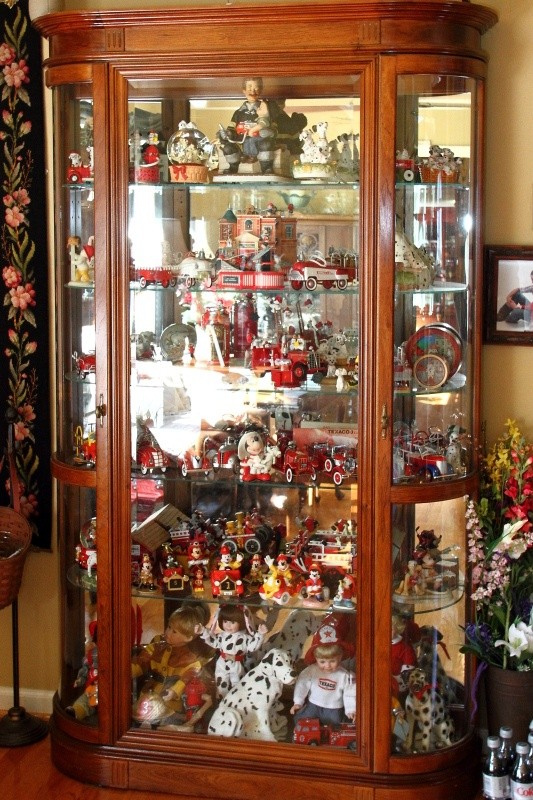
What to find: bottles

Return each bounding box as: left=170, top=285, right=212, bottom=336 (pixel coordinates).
left=482, top=725, right=533, bottom=800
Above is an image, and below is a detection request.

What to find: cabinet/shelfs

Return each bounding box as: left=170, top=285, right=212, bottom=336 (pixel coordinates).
left=23, top=0, right=510, bottom=800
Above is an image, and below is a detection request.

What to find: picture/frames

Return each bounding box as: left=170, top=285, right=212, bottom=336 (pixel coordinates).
left=481, top=244, right=533, bottom=347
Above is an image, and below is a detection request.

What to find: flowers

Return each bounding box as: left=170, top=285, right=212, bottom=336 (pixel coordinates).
left=0, top=0, right=38, bottom=521
left=464, top=418, right=533, bottom=673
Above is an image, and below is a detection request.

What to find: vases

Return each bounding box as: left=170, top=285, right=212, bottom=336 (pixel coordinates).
left=480, top=664, right=533, bottom=750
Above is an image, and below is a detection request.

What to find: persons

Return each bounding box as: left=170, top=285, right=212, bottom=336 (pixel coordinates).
left=64, top=603, right=267, bottom=724
left=498, top=271, right=533, bottom=324
left=392, top=613, right=417, bottom=699
left=291, top=619, right=358, bottom=729
left=216, top=76, right=281, bottom=174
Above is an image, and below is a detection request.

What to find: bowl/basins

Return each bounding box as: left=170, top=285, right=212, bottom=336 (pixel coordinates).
left=406, top=325, right=462, bottom=388
